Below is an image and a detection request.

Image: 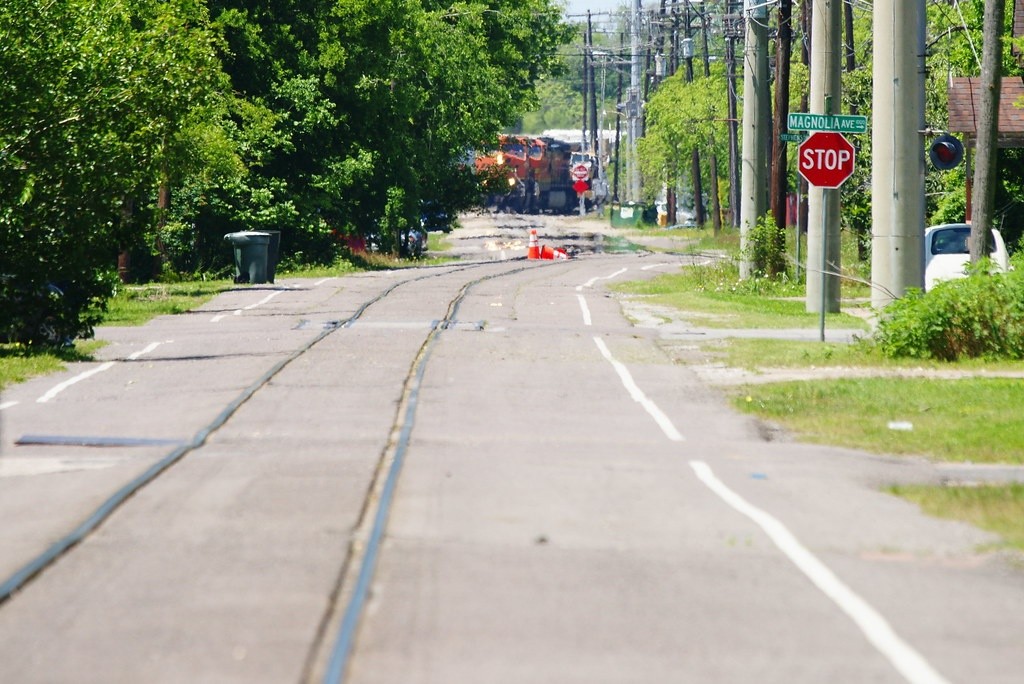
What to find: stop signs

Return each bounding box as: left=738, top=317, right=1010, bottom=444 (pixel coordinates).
left=571, top=164, right=590, bottom=181
left=798, top=131, right=855, bottom=189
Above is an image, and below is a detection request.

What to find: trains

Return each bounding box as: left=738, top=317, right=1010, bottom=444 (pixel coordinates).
left=470, top=135, right=604, bottom=215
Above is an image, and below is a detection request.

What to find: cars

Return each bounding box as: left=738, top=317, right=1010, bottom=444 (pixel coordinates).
left=925, top=223, right=1012, bottom=292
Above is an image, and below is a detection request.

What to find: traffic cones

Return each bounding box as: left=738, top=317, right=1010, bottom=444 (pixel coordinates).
left=527, top=229, right=542, bottom=260
left=540, top=244, right=569, bottom=260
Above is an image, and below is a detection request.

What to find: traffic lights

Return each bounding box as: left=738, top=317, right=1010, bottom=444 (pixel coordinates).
left=927, top=134, right=964, bottom=171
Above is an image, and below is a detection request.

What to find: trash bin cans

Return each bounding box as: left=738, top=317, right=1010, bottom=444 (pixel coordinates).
left=261, top=231, right=281, bottom=283
left=224, top=232, right=271, bottom=286
left=612, top=202, right=644, bottom=228
